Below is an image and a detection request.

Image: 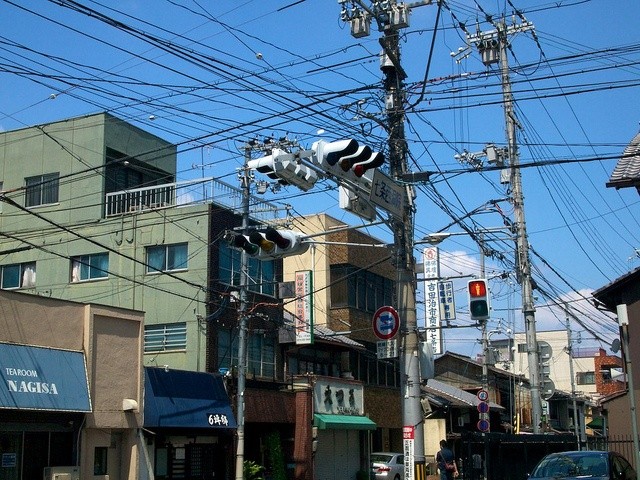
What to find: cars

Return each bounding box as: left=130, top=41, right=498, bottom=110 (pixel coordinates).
left=370, top=452, right=404, bottom=480
left=525, top=451, right=637, bottom=479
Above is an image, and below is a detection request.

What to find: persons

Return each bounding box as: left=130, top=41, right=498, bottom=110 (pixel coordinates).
left=436, top=440, right=459, bottom=480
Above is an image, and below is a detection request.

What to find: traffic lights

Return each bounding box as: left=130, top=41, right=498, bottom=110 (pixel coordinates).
left=467, top=278, right=490, bottom=320
left=234, top=227, right=310, bottom=261
left=310, top=139, right=385, bottom=195
left=247, top=147, right=319, bottom=192
